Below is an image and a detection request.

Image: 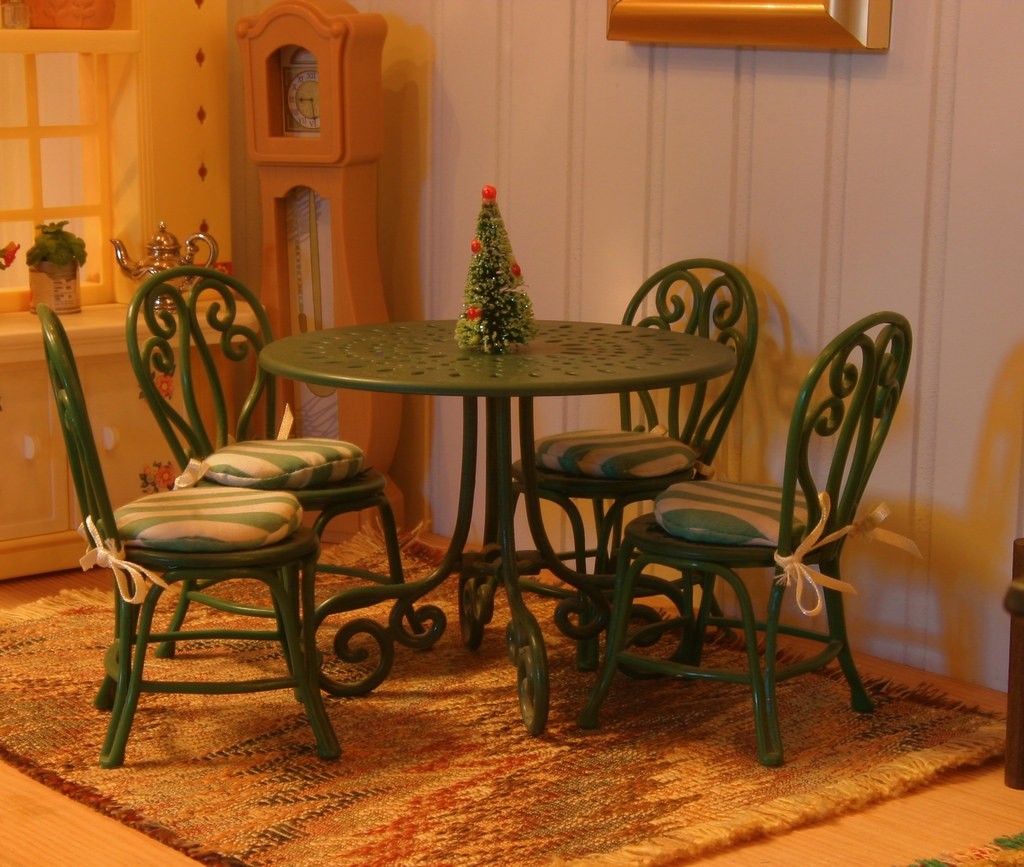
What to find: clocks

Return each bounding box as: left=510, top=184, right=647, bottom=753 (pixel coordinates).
left=280, top=63, right=321, bottom=138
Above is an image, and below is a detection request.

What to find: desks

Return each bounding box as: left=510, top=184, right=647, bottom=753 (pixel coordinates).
left=257, top=317, right=739, bottom=736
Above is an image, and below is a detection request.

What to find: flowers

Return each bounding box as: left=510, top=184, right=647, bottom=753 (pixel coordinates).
left=0, top=241, right=20, bottom=269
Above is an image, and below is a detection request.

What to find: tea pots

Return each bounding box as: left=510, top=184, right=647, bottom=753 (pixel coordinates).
left=108, top=217, right=219, bottom=317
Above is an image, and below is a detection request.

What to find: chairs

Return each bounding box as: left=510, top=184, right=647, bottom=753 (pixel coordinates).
left=494, top=257, right=760, bottom=672
left=573, top=311, right=912, bottom=767
left=126, top=267, right=433, bottom=700
left=42, top=308, right=343, bottom=768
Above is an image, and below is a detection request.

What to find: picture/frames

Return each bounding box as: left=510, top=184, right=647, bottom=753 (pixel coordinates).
left=606, top=0, right=892, bottom=50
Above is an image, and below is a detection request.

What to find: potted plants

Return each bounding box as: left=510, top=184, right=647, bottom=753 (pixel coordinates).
left=27, top=221, right=87, bottom=316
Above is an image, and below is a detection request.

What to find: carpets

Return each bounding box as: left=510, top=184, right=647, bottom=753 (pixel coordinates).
left=0, top=519, right=1009, bottom=867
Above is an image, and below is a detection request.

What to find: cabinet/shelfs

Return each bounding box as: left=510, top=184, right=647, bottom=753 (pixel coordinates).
left=0, top=350, right=196, bottom=540
